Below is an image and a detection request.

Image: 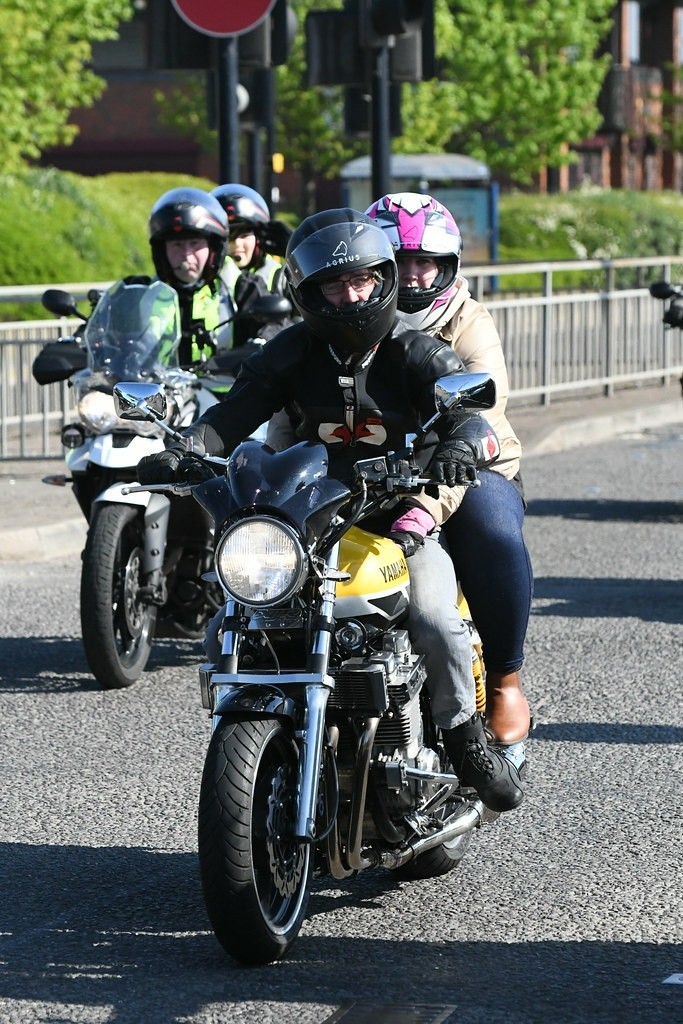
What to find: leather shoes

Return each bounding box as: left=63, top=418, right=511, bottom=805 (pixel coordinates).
left=486, top=667, right=531, bottom=747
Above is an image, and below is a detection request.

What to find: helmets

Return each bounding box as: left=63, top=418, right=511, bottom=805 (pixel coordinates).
left=365, top=192, right=462, bottom=334
left=283, top=208, right=399, bottom=352
left=209, top=183, right=270, bottom=272
left=149, top=187, right=229, bottom=293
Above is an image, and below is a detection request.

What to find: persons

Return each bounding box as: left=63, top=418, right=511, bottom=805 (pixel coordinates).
left=207, top=182, right=288, bottom=295
left=139, top=205, right=524, bottom=811
left=363, top=191, right=533, bottom=744
left=32, top=186, right=292, bottom=480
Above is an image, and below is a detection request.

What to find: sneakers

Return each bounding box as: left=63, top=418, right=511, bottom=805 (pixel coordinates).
left=443, top=718, right=525, bottom=812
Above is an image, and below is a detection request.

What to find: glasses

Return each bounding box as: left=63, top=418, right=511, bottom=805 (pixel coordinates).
left=321, top=273, right=375, bottom=296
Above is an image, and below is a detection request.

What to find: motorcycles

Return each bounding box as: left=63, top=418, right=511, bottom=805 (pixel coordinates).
left=39, top=271, right=279, bottom=687
left=108, top=365, right=526, bottom=966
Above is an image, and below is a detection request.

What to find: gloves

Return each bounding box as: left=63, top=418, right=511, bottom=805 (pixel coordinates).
left=137, top=447, right=184, bottom=498
left=424, top=447, right=477, bottom=502
